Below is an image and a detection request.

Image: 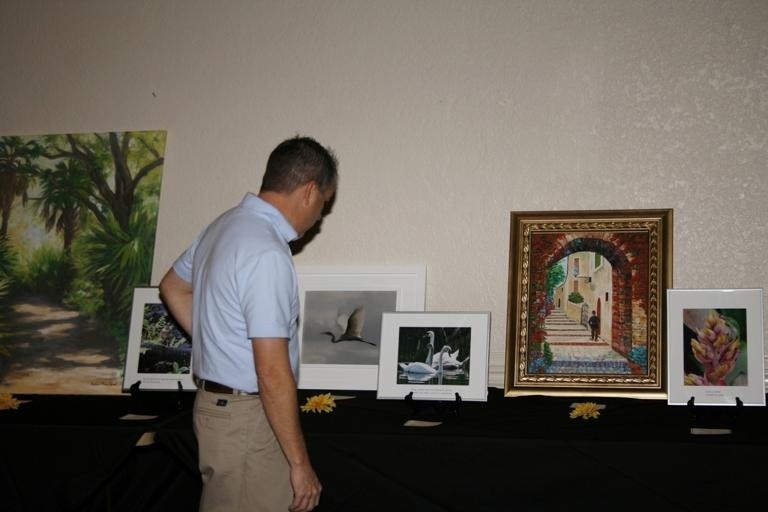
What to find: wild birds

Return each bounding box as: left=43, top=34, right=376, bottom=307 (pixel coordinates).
left=320, top=305, right=378, bottom=347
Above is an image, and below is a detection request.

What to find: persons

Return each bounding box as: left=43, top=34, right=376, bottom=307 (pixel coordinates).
left=159, top=137, right=336, bottom=512
left=589, top=311, right=600, bottom=341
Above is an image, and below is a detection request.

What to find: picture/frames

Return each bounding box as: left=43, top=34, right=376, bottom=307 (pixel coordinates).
left=295, top=265, right=426, bottom=391
left=666, top=286, right=765, bottom=405
left=125, top=288, right=196, bottom=391
left=378, top=313, right=489, bottom=400
left=504, top=209, right=673, bottom=397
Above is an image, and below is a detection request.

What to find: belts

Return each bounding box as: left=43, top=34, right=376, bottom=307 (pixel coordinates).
left=192, top=374, right=259, bottom=395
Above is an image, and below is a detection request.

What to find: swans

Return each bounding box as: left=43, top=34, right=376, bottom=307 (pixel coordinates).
left=422, top=330, right=460, bottom=360
left=424, top=344, right=469, bottom=375
left=399, top=345, right=451, bottom=374
